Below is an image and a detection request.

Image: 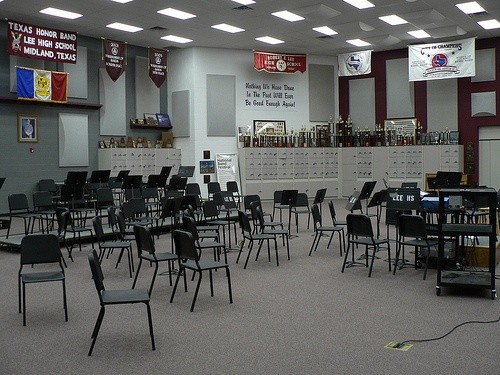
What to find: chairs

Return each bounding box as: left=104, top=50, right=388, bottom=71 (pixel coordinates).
left=0, top=179, right=444, bottom=356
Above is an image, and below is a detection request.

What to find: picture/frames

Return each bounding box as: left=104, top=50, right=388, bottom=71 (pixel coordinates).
left=18, top=114, right=39, bottom=142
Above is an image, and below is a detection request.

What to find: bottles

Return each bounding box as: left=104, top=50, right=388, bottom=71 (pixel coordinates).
left=260, top=114, right=450, bottom=147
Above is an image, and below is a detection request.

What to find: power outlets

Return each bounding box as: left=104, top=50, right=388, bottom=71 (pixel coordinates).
left=384, top=340, right=413, bottom=351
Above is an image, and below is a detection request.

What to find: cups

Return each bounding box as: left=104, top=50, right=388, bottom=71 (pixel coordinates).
left=253, top=137, right=259, bottom=147
left=244, top=136, right=251, bottom=147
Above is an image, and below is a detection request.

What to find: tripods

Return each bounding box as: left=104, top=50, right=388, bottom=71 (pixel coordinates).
left=279, top=190, right=299, bottom=239
left=345, top=179, right=422, bottom=271
left=158, top=198, right=183, bottom=275
left=312, top=188, right=329, bottom=236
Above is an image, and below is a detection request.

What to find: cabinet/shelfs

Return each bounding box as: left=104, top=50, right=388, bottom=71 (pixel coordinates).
left=340, top=145, right=464, bottom=199
left=238, top=148, right=339, bottom=200
left=98, top=149, right=181, bottom=183
